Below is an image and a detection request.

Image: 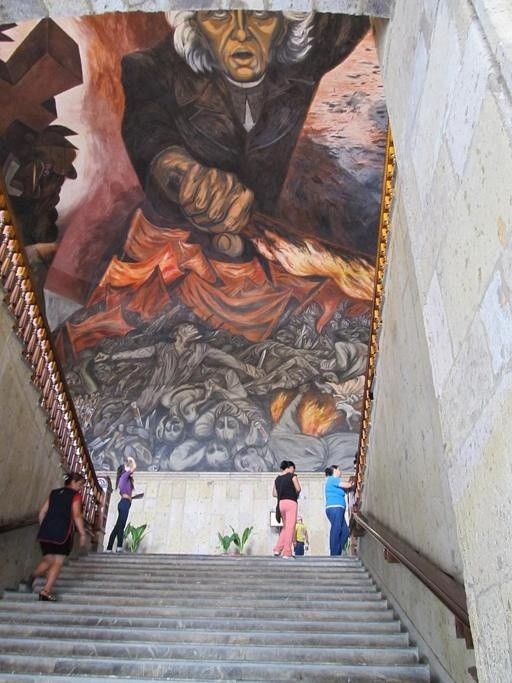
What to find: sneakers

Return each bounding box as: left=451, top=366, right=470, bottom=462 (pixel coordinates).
left=106, top=550, right=112, bottom=553
left=274, top=551, right=281, bottom=556
left=116, top=547, right=128, bottom=554
left=283, top=555, right=296, bottom=561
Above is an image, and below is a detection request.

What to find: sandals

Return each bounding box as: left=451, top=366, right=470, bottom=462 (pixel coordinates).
left=38, top=589, right=57, bottom=601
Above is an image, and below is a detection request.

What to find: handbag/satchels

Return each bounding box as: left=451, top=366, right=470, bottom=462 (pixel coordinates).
left=276, top=496, right=282, bottom=523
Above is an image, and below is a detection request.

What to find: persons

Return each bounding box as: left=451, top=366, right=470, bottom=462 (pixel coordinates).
left=25, top=474, right=87, bottom=600
left=293, top=515, right=310, bottom=555
left=325, top=465, right=356, bottom=556
left=272, top=460, right=302, bottom=560
left=104, top=458, right=136, bottom=554
